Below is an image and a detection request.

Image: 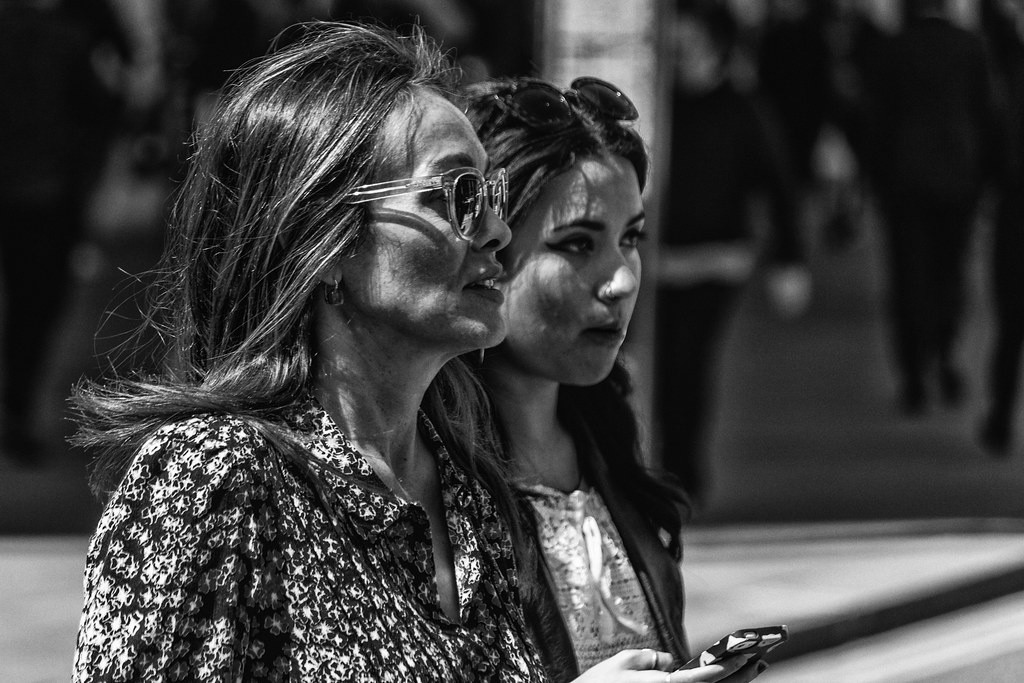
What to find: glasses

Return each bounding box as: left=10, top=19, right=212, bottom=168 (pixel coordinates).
left=478, top=76, right=639, bottom=143
left=314, top=166, right=509, bottom=241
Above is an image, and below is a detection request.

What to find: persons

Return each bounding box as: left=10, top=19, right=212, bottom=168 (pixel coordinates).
left=660, top=0, right=1024, bottom=491
left=72, top=30, right=768, bottom=683
left=456, top=80, right=690, bottom=672
left=0, top=0, right=537, bottom=462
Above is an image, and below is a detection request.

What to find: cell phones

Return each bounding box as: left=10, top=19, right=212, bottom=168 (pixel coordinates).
left=679, top=624, right=788, bottom=673
